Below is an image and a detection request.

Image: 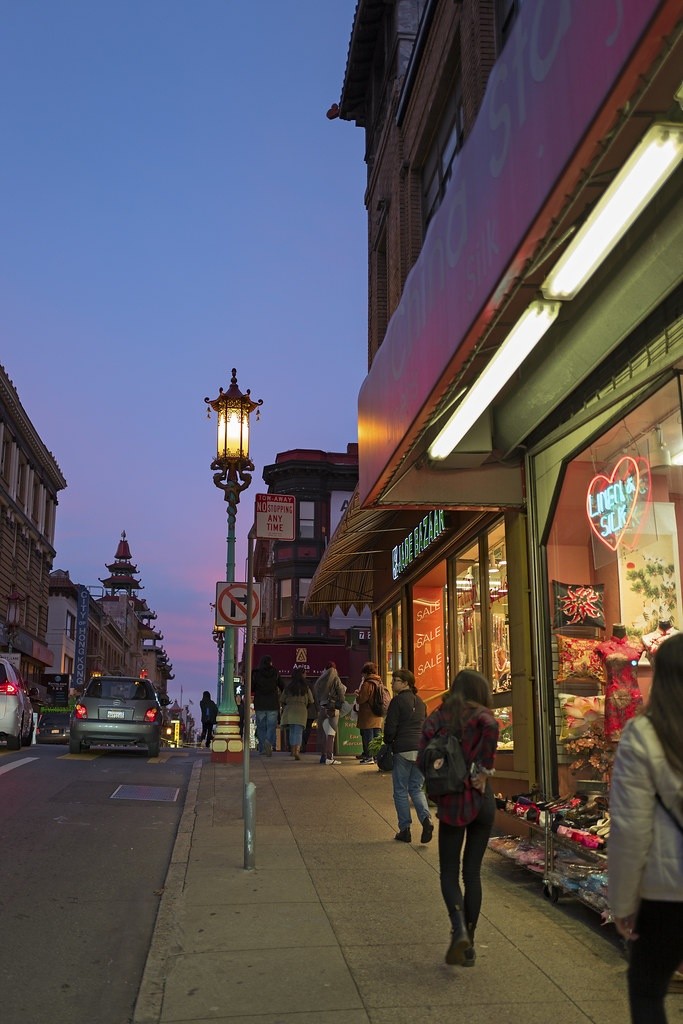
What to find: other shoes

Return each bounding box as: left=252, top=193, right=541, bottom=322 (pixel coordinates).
left=258, top=741, right=273, bottom=757
left=421, top=817, right=434, bottom=843
left=396, top=827, right=411, bottom=842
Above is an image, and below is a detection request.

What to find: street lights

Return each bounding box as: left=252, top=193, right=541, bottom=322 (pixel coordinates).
left=205, top=367, right=264, bottom=766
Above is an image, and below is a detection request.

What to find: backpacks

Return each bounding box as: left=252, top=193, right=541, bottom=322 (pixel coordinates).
left=364, top=677, right=391, bottom=717
left=419, top=706, right=483, bottom=798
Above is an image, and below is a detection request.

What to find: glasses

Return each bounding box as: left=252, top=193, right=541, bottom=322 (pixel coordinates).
left=394, top=678, right=403, bottom=682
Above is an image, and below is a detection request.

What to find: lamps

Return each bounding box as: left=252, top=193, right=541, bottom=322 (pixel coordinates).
left=539, top=119, right=683, bottom=301
left=425, top=301, right=561, bottom=462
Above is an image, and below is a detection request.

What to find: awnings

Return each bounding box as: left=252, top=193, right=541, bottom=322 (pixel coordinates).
left=358, top=0, right=683, bottom=511
left=304, top=482, right=409, bottom=616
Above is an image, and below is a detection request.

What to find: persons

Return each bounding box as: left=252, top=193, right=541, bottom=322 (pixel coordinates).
left=235, top=694, right=244, bottom=738
left=200, top=691, right=218, bottom=748
left=354, top=663, right=382, bottom=764
left=418, top=669, right=499, bottom=966
left=595, top=624, right=645, bottom=741
left=384, top=670, right=435, bottom=844
left=608, top=632, right=683, bottom=1023
left=251, top=656, right=314, bottom=759
left=314, top=662, right=345, bottom=764
left=640, top=618, right=681, bottom=671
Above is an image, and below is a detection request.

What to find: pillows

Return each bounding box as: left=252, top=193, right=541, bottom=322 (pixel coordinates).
left=551, top=579, right=607, bottom=631
left=558, top=692, right=621, bottom=743
left=554, top=634, right=609, bottom=684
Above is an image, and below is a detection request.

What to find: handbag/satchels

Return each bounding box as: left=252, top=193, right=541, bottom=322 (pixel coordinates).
left=377, top=743, right=394, bottom=771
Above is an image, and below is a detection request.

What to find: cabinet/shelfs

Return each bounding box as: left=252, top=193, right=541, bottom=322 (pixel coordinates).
left=554, top=623, right=610, bottom=744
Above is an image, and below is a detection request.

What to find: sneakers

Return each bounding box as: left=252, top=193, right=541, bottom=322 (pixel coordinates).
left=356, top=752, right=365, bottom=759
left=360, top=757, right=377, bottom=764
left=325, top=758, right=342, bottom=765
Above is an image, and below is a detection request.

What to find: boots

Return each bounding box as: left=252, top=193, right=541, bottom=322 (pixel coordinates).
left=290, top=745, right=296, bottom=757
left=446, top=910, right=477, bottom=967
left=294, top=745, right=301, bottom=760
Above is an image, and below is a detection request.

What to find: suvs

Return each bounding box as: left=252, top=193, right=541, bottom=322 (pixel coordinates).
left=69, top=675, right=162, bottom=757
left=0, top=658, right=39, bottom=749
left=36, top=712, right=71, bottom=745
